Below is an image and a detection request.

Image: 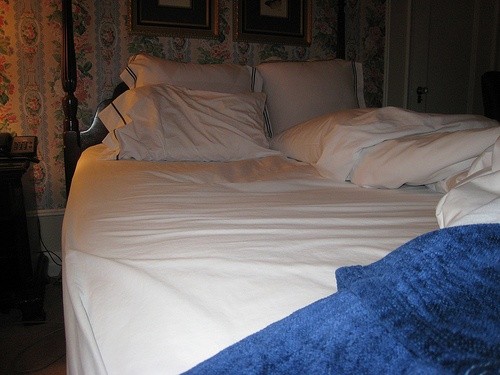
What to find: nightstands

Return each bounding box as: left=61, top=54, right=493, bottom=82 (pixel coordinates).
left=2, top=161, right=50, bottom=327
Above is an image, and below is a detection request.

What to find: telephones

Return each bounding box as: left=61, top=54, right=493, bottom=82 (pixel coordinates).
left=0, top=132, right=41, bottom=164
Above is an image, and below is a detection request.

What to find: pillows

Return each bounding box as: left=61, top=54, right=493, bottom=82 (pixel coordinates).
left=98, top=53, right=369, bottom=162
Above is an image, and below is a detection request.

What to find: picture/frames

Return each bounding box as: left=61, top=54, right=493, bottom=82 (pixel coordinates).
left=127, top=0, right=220, bottom=40
left=232, top=1, right=313, bottom=48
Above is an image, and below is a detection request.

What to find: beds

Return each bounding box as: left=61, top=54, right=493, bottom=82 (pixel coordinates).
left=60, top=0, right=499, bottom=375
